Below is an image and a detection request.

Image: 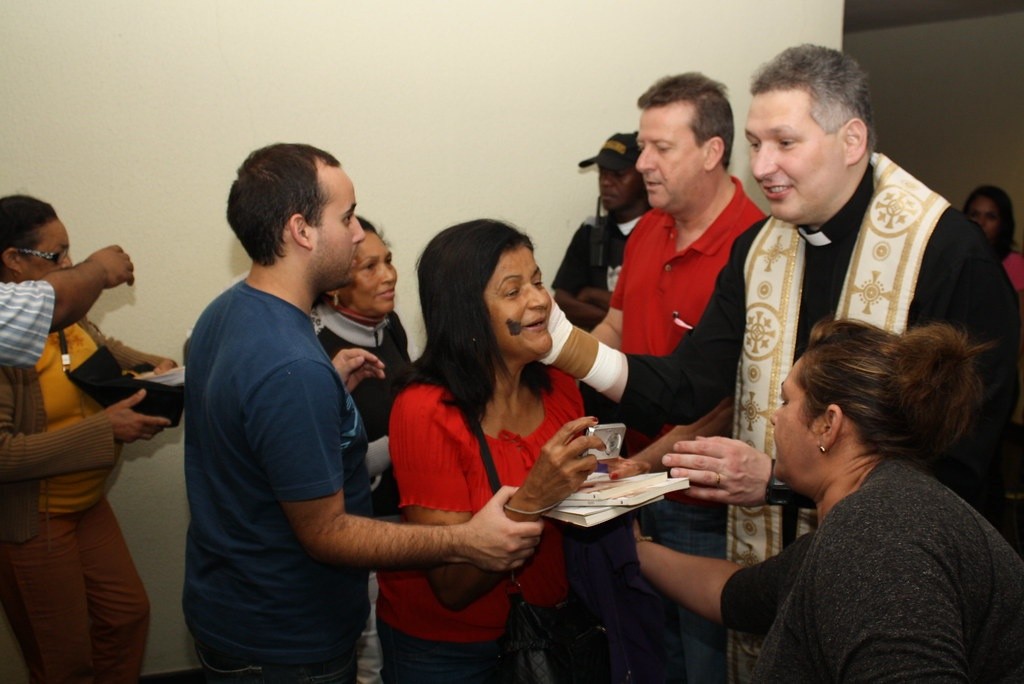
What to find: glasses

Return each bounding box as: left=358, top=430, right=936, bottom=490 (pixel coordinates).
left=15, top=248, right=68, bottom=264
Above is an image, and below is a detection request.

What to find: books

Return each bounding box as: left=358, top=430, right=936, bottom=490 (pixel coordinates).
left=544, top=470, right=690, bottom=528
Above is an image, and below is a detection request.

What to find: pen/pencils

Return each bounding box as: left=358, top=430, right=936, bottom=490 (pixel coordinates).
left=672, top=310, right=678, bottom=318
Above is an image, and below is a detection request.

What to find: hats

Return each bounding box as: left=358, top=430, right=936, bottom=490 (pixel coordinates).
left=579, top=133, right=642, bottom=171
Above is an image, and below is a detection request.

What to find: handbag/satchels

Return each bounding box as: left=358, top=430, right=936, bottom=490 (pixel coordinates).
left=502, top=592, right=611, bottom=684
left=59, top=323, right=185, bottom=427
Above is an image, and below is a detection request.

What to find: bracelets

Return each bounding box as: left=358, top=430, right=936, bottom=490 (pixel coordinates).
left=503, top=503, right=561, bottom=515
left=634, top=534, right=652, bottom=543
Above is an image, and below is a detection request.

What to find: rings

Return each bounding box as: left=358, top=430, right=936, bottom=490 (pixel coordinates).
left=717, top=474, right=721, bottom=487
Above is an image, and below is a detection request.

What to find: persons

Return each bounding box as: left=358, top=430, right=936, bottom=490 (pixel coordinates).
left=587, top=72, right=770, bottom=683
left=554, top=127, right=651, bottom=423
left=181, top=144, right=547, bottom=684
left=960, top=184, right=1024, bottom=307
left=632, top=316, right=1024, bottom=683
left=375, top=219, right=640, bottom=684
left=536, top=46, right=1024, bottom=684
left=0, top=245, right=135, bottom=684
left=311, top=214, right=415, bottom=684
left=0, top=195, right=176, bottom=684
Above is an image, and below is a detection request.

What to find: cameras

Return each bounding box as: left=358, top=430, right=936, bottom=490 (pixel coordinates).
left=581, top=423, right=628, bottom=460
